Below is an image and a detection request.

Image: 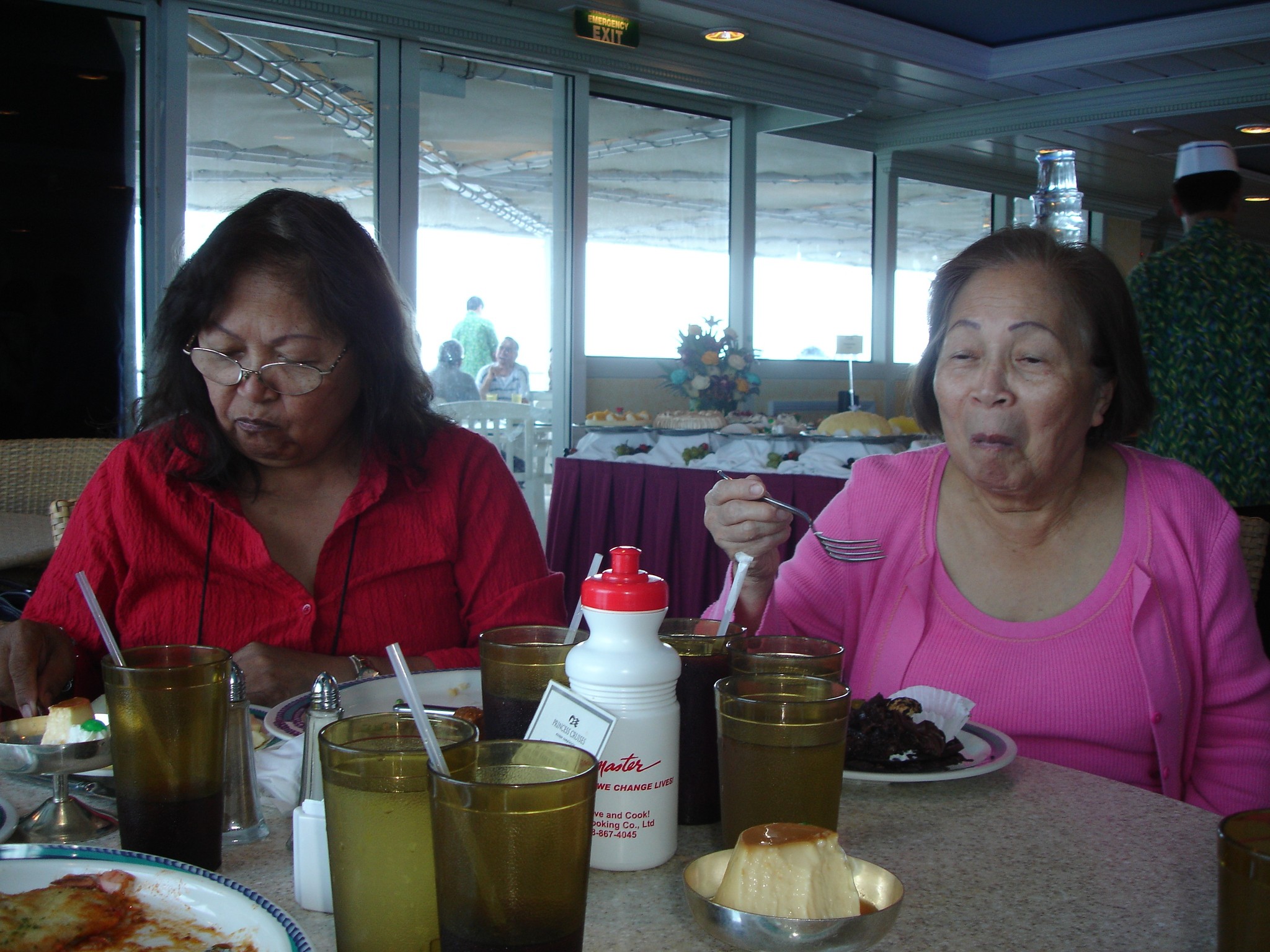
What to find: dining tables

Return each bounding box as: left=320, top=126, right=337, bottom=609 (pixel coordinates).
left=545, top=447, right=847, bottom=620
left=70, top=748, right=1229, bottom=952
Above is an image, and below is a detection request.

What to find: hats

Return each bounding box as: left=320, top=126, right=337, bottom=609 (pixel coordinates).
left=1173, top=141, right=1243, bottom=183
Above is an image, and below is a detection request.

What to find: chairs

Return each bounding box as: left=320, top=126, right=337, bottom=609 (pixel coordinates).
left=435, top=397, right=550, bottom=540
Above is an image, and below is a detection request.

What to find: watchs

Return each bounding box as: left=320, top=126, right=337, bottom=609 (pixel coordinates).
left=346, top=650, right=378, bottom=681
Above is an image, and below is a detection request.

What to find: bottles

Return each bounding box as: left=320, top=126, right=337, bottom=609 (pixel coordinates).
left=285, top=672, right=344, bottom=849
left=565, top=546, right=681, bottom=871
left=220, top=660, right=271, bottom=845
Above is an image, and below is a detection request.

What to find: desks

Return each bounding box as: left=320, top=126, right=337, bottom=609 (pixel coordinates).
left=460, top=418, right=553, bottom=534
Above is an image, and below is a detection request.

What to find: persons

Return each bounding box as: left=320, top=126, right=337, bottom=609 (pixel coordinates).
left=423, top=339, right=480, bottom=403
left=694, top=228, right=1269, bottom=841
left=471, top=335, right=532, bottom=400
left=1127, top=136, right=1270, bottom=529
left=0, top=186, right=572, bottom=719
left=451, top=296, right=500, bottom=392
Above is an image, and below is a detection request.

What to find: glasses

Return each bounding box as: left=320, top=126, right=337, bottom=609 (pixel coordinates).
left=182, top=331, right=350, bottom=396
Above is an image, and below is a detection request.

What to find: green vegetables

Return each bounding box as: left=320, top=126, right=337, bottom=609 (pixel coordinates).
left=0, top=917, right=83, bottom=952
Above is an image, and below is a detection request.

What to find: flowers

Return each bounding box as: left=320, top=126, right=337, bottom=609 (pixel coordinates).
left=654, top=314, right=761, bottom=409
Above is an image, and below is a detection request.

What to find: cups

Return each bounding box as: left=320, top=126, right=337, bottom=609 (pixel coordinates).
left=1216, top=808, right=1270, bottom=952
left=714, top=635, right=852, bottom=850
left=479, top=625, right=590, bottom=850
left=318, top=712, right=480, bottom=952
left=426, top=739, right=599, bottom=952
left=101, top=645, right=233, bottom=872
left=511, top=393, right=522, bottom=403
left=486, top=393, right=498, bottom=401
left=658, top=618, right=747, bottom=826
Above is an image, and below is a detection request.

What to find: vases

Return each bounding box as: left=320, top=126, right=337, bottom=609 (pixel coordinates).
left=691, top=394, right=739, bottom=414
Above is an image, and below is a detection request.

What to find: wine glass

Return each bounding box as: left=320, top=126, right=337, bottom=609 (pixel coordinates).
left=0, top=713, right=119, bottom=843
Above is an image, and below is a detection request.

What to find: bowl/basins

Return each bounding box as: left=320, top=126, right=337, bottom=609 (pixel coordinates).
left=682, top=848, right=905, bottom=952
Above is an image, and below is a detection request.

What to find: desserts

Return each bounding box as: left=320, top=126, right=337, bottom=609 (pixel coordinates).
left=712, top=820, right=860, bottom=919
left=38, top=696, right=95, bottom=744
left=64, top=718, right=110, bottom=744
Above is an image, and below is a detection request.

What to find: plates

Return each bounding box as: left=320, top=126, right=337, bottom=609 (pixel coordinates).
left=0, top=796, right=19, bottom=844
left=843, top=719, right=1018, bottom=784
left=263, top=667, right=482, bottom=741
left=71, top=704, right=285, bottom=775
left=0, top=843, right=317, bottom=952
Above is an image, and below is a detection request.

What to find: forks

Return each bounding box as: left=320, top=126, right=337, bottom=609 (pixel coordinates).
left=717, top=469, right=888, bottom=562
